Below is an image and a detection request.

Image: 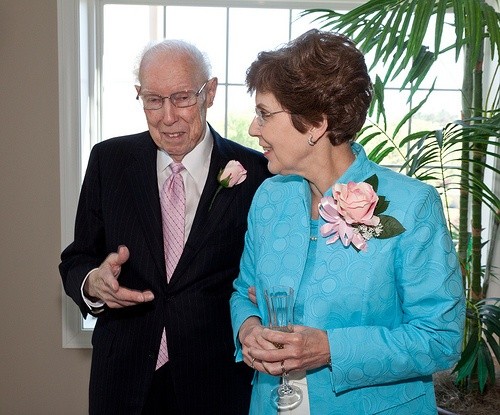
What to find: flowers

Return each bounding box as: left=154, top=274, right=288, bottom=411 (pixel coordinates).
left=331, top=173, right=406, bottom=241
left=209, top=160, right=247, bottom=211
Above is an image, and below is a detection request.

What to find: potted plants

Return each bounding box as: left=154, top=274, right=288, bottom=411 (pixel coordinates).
left=295, top=0, right=500, bottom=415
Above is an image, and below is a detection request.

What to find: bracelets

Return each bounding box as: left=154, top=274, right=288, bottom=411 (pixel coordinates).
left=328, top=356, right=332, bottom=365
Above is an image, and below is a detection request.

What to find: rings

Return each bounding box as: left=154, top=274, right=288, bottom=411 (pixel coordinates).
left=281, top=361, right=286, bottom=372
left=282, top=372, right=289, bottom=377
left=251, top=359, right=256, bottom=365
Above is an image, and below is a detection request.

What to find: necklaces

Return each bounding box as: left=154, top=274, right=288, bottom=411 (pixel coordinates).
left=310, top=237, right=317, bottom=240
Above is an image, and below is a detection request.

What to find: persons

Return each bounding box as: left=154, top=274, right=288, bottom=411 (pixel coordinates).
left=58, top=40, right=276, bottom=415
left=228, top=28, right=467, bottom=415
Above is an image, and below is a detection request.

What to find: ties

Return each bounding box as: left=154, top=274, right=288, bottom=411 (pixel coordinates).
left=154, top=161, right=185, bottom=370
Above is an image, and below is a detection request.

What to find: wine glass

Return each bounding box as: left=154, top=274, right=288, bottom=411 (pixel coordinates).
left=264, top=284, right=302, bottom=410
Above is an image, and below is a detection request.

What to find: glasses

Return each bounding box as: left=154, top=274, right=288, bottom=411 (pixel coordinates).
left=255, top=107, right=289, bottom=124
left=136, top=79, right=209, bottom=110
